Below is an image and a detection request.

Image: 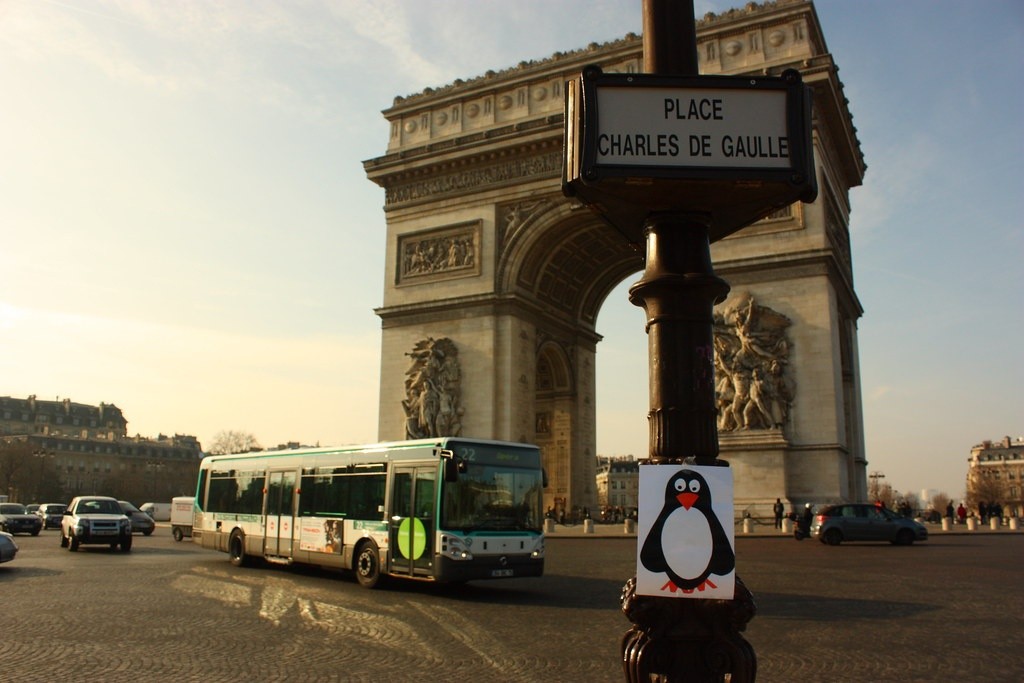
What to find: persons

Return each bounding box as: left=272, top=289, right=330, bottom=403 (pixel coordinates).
left=503, top=199, right=545, bottom=241
left=579, top=505, right=625, bottom=524
left=325, top=521, right=341, bottom=552
left=715, top=296, right=792, bottom=432
left=957, top=503, right=966, bottom=520
left=894, top=496, right=916, bottom=521
left=774, top=498, right=784, bottom=529
left=946, top=500, right=955, bottom=524
left=802, top=503, right=814, bottom=522
left=876, top=499, right=885, bottom=507
left=548, top=506, right=566, bottom=524
left=403, top=341, right=460, bottom=441
left=407, top=236, right=473, bottom=275
left=978, top=501, right=1004, bottom=524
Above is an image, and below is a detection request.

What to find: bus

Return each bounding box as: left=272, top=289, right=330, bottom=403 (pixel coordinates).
left=191, top=436, right=548, bottom=594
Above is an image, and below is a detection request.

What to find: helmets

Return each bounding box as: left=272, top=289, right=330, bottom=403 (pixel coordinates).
left=805, top=502, right=812, bottom=509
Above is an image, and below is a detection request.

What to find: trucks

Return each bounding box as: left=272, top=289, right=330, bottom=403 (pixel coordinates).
left=138, top=503, right=172, bottom=521
left=170, top=496, right=197, bottom=541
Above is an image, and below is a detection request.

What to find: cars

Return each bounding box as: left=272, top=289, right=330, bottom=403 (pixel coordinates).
left=118, top=500, right=155, bottom=535
left=35, top=503, right=68, bottom=530
left=0, top=530, right=19, bottom=564
left=26, top=504, right=41, bottom=515
left=0, top=503, right=42, bottom=536
left=809, top=503, right=929, bottom=547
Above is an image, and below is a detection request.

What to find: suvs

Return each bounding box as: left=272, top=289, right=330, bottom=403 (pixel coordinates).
left=60, top=496, right=132, bottom=553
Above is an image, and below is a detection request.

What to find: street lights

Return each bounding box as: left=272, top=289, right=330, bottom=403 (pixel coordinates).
left=868, top=471, right=885, bottom=497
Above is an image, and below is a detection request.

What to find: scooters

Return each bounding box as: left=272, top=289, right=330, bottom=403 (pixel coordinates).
left=794, top=515, right=811, bottom=541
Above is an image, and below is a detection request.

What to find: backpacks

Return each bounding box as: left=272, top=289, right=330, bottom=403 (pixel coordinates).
left=775, top=503, right=782, bottom=513
